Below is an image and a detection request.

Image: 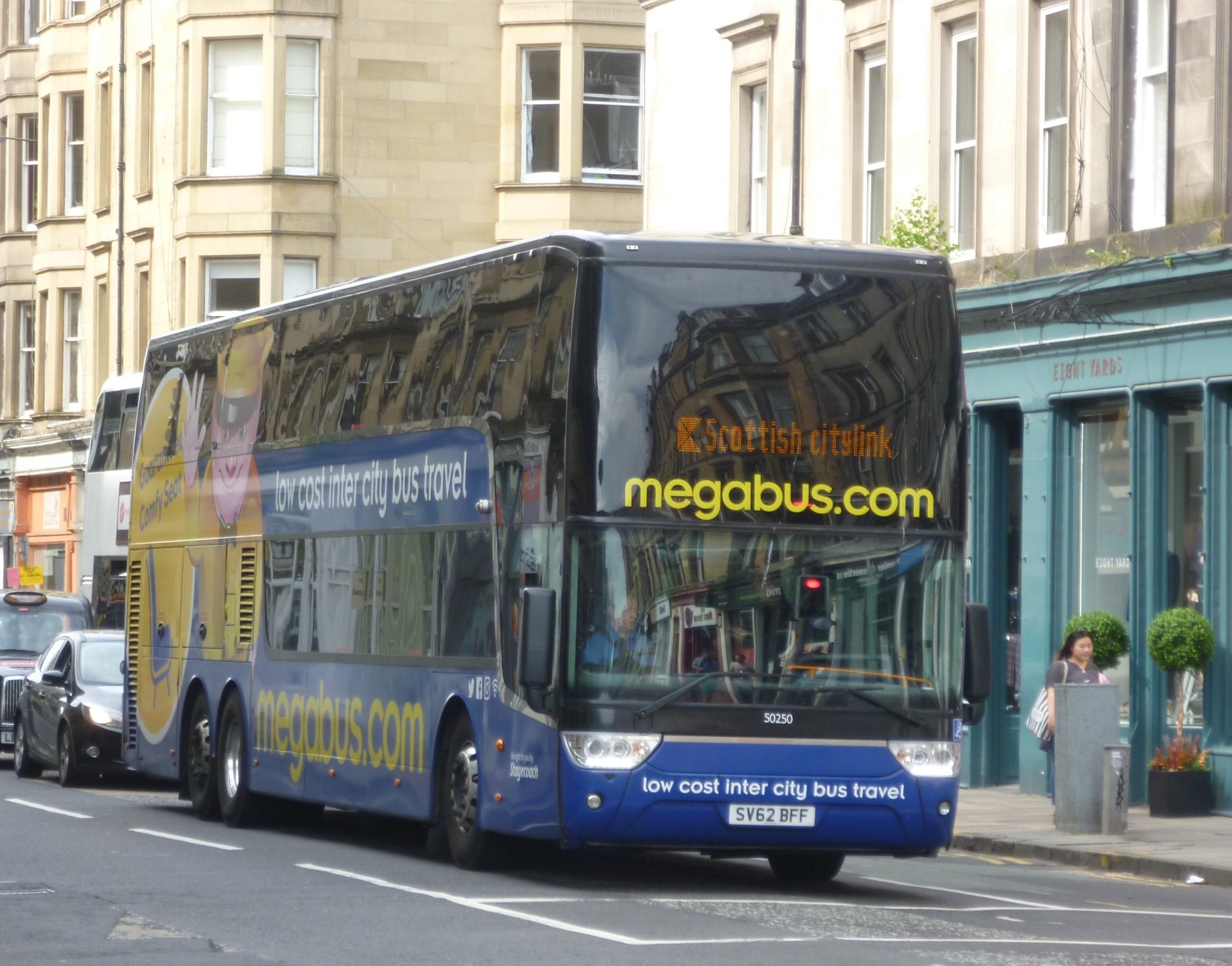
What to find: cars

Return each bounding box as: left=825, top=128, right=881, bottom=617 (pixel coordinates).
left=14, top=629, right=126, bottom=788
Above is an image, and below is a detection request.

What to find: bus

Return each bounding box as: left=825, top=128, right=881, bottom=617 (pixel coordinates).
left=80, top=370, right=142, bottom=629
left=120, top=231, right=995, bottom=879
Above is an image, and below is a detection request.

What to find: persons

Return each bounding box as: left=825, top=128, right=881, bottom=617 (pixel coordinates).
left=581, top=589, right=663, bottom=674
left=729, top=655, right=757, bottom=680
left=1040, top=630, right=1100, bottom=807
left=691, top=651, right=712, bottom=675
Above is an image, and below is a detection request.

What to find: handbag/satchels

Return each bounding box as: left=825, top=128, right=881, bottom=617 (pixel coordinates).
left=1025, top=687, right=1052, bottom=741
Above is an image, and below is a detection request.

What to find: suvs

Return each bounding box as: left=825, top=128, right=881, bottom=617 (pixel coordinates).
left=0, top=587, right=98, bottom=753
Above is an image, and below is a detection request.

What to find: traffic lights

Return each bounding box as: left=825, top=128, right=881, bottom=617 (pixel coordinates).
left=795, top=575, right=830, bottom=621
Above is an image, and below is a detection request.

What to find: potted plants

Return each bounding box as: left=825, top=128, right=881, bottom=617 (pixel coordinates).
left=1147, top=607, right=1217, bottom=815
